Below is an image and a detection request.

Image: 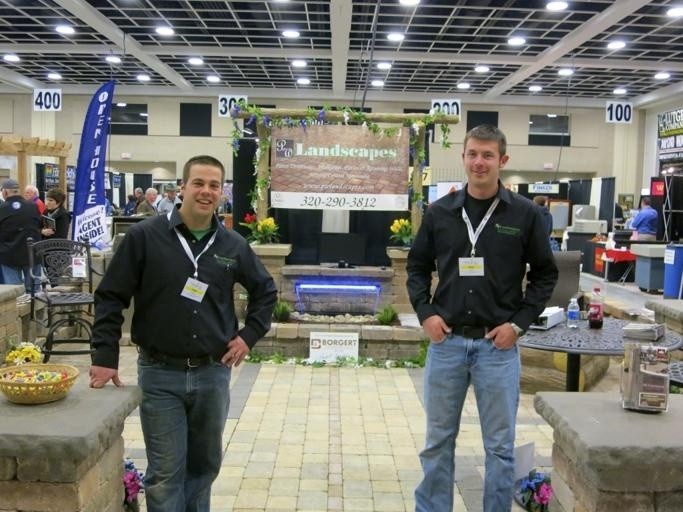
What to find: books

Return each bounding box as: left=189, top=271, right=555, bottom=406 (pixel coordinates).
left=621, top=323, right=665, bottom=341
left=618, top=342, right=669, bottom=413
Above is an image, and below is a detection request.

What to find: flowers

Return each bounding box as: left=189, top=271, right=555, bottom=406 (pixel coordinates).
left=513, top=469, right=553, bottom=512
left=388, top=218, right=416, bottom=245
left=238, top=214, right=280, bottom=245
left=123, top=457, right=157, bottom=512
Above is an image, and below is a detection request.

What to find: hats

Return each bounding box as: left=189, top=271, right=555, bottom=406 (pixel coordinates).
left=165, top=183, right=178, bottom=191
left=0, top=179, right=19, bottom=191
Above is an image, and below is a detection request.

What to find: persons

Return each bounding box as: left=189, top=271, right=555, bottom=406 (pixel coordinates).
left=531, top=194, right=553, bottom=237
left=85, top=154, right=280, bottom=511
left=1, top=171, right=182, bottom=279
left=628, top=195, right=658, bottom=242
left=402, top=127, right=559, bottom=512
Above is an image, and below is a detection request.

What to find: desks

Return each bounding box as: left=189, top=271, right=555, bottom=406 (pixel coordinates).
left=517, top=316, right=682, bottom=392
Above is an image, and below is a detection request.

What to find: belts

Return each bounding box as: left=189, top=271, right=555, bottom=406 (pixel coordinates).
left=141, top=350, right=209, bottom=368
left=453, top=325, right=493, bottom=339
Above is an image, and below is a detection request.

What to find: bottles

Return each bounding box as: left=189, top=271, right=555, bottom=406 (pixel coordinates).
left=588, top=288, right=604, bottom=330
left=567, top=297, right=580, bottom=329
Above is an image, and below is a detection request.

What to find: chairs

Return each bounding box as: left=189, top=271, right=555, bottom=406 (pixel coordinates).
left=26, top=236, right=105, bottom=364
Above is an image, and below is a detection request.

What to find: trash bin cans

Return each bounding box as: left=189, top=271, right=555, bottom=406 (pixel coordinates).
left=663, top=244, right=683, bottom=300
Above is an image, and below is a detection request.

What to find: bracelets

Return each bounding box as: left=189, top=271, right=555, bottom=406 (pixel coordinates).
left=511, top=323, right=524, bottom=338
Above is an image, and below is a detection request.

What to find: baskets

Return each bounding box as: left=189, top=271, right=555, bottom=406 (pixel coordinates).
left=0, top=363, right=79, bottom=405
